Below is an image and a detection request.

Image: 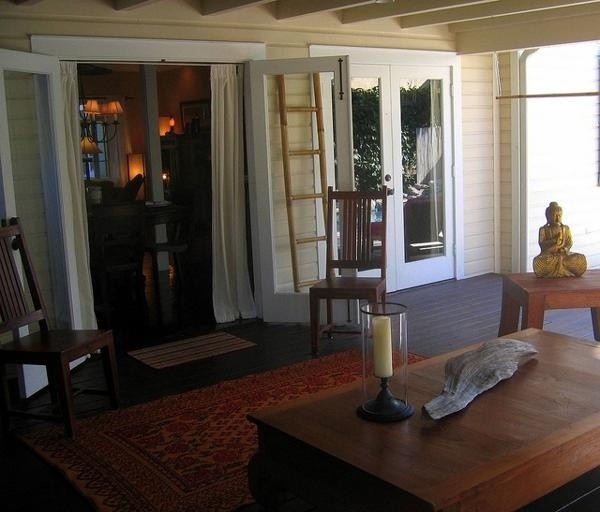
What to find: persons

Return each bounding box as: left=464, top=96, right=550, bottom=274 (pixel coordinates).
left=532, top=202, right=588, bottom=279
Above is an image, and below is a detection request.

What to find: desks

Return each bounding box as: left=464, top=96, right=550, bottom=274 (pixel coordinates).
left=497, top=270, right=600, bottom=341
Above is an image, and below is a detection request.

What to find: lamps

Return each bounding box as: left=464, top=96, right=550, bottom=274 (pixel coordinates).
left=80, top=97, right=124, bottom=183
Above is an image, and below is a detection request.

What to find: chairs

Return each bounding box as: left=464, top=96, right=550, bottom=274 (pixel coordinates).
left=1, top=216, right=119, bottom=432
left=308, top=185, right=387, bottom=355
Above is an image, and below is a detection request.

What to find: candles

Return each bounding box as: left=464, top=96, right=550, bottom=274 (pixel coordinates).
left=355, top=301, right=415, bottom=421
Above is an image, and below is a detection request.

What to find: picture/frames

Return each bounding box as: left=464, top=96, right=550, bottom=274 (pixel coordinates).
left=180, top=100, right=210, bottom=130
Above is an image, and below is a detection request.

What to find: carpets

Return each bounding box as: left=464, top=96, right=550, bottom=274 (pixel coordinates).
left=126, top=332, right=252, bottom=369
left=20, top=346, right=429, bottom=511
